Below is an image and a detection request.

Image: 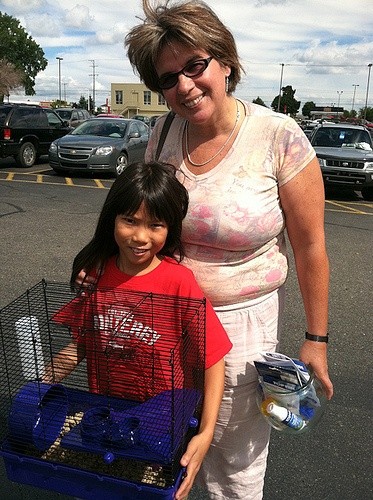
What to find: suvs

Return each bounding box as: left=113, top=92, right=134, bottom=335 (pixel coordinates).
left=53, top=108, right=97, bottom=132
left=306, top=118, right=373, bottom=201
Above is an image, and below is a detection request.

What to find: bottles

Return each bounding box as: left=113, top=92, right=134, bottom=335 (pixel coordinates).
left=266, top=403, right=305, bottom=431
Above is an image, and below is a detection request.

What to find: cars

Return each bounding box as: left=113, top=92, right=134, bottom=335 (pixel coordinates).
left=96, top=114, right=124, bottom=124
left=300, top=118, right=336, bottom=139
left=132, top=115, right=151, bottom=127
left=150, top=115, right=161, bottom=126
left=47, top=118, right=153, bottom=178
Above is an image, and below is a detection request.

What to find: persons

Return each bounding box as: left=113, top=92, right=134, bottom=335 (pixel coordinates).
left=42, top=160, right=232, bottom=500
left=72, top=0, right=333, bottom=500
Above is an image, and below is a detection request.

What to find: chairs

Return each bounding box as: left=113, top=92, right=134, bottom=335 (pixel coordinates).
left=317, top=133, right=368, bottom=148
left=101, top=122, right=123, bottom=135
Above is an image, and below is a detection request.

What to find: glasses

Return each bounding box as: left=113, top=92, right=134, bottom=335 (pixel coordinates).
left=156, top=56, right=214, bottom=90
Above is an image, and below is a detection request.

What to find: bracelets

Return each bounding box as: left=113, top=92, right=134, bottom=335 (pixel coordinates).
left=305, top=332, right=329, bottom=343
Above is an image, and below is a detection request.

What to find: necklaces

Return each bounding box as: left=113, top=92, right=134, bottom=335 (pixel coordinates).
left=185, top=102, right=241, bottom=166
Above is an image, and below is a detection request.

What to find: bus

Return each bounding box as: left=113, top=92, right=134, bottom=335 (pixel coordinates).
left=0, top=102, right=75, bottom=168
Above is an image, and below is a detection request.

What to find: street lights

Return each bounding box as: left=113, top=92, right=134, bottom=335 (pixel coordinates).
left=336, top=90, right=344, bottom=116
left=56, top=57, right=65, bottom=106
left=351, top=84, right=360, bottom=112
